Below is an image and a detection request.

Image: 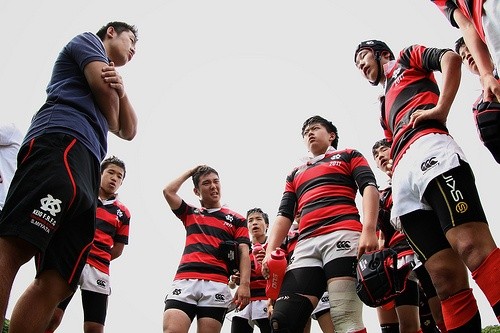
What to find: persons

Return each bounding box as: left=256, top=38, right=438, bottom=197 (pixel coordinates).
left=162, top=0, right=500, bottom=333
left=44, top=155, right=131, bottom=333
left=0, top=22, right=138, bottom=333
left=0, top=122, right=25, bottom=212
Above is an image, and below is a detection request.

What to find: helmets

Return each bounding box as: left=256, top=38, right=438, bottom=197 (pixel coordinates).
left=355, top=249, right=416, bottom=307
left=476, top=98, right=500, bottom=165
